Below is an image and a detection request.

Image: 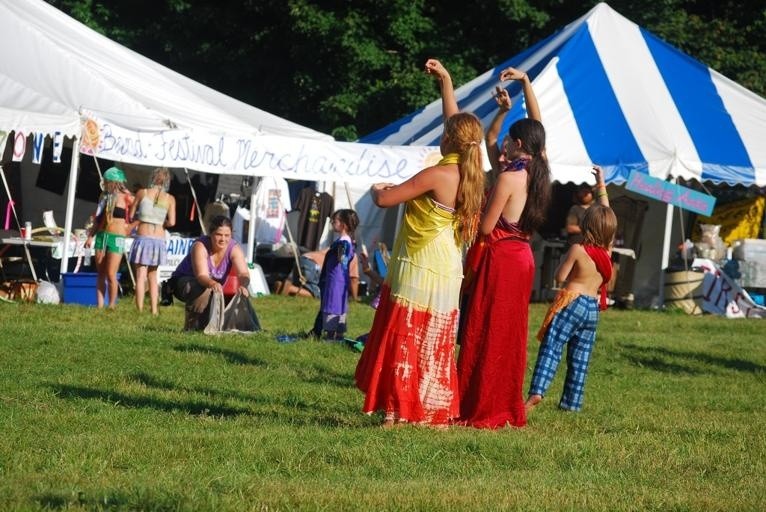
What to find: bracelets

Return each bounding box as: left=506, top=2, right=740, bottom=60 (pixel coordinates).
left=597, top=190, right=609, bottom=195
left=519, top=73, right=527, bottom=80
left=597, top=183, right=606, bottom=188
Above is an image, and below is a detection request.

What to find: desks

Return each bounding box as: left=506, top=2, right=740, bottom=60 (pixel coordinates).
left=534, top=237, right=636, bottom=305
left=0, top=228, right=205, bottom=301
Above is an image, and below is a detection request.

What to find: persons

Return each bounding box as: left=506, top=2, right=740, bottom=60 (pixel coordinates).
left=305, top=209, right=360, bottom=341
left=83, top=166, right=141, bottom=310
left=354, top=58, right=485, bottom=426
left=170, top=215, right=251, bottom=332
left=454, top=84, right=513, bottom=348
left=566, top=184, right=597, bottom=245
left=453, top=67, right=550, bottom=428
left=523, top=167, right=616, bottom=412
left=126, top=169, right=176, bottom=317
left=274, top=245, right=359, bottom=299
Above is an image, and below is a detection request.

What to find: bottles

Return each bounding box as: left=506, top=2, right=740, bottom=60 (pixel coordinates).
left=726, top=242, right=733, bottom=260
left=24, top=221, right=31, bottom=240
left=709, top=245, right=716, bottom=259
left=614, top=231, right=624, bottom=248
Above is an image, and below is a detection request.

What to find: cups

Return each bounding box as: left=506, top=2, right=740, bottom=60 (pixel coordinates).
left=74, top=229, right=86, bottom=240
left=19, top=228, right=25, bottom=237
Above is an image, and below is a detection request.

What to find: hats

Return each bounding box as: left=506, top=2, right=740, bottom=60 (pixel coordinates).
left=103, top=167, right=127, bottom=182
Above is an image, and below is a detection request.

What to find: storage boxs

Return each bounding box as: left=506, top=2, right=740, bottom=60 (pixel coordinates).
left=59, top=270, right=123, bottom=306
